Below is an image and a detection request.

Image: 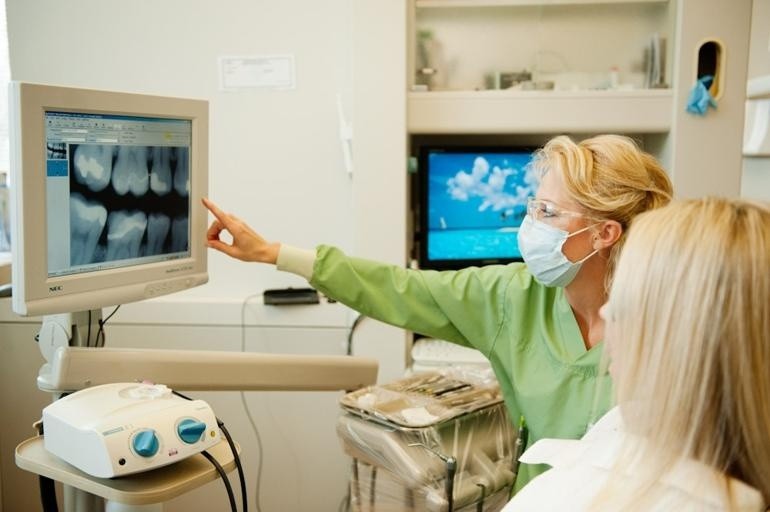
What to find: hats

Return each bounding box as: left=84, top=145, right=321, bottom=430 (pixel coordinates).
left=527, top=197, right=606, bottom=223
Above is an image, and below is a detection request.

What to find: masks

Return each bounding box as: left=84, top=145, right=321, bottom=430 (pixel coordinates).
left=517, top=215, right=607, bottom=288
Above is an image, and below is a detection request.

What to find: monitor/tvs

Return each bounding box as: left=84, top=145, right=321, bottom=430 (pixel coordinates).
left=8, top=80, right=210, bottom=321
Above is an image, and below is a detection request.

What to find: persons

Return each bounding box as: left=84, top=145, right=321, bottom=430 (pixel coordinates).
left=202, top=132, right=672, bottom=503
left=500, top=200, right=770, bottom=512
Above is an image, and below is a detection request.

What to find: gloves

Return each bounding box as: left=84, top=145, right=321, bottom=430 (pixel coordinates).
left=685, top=74, right=719, bottom=116
